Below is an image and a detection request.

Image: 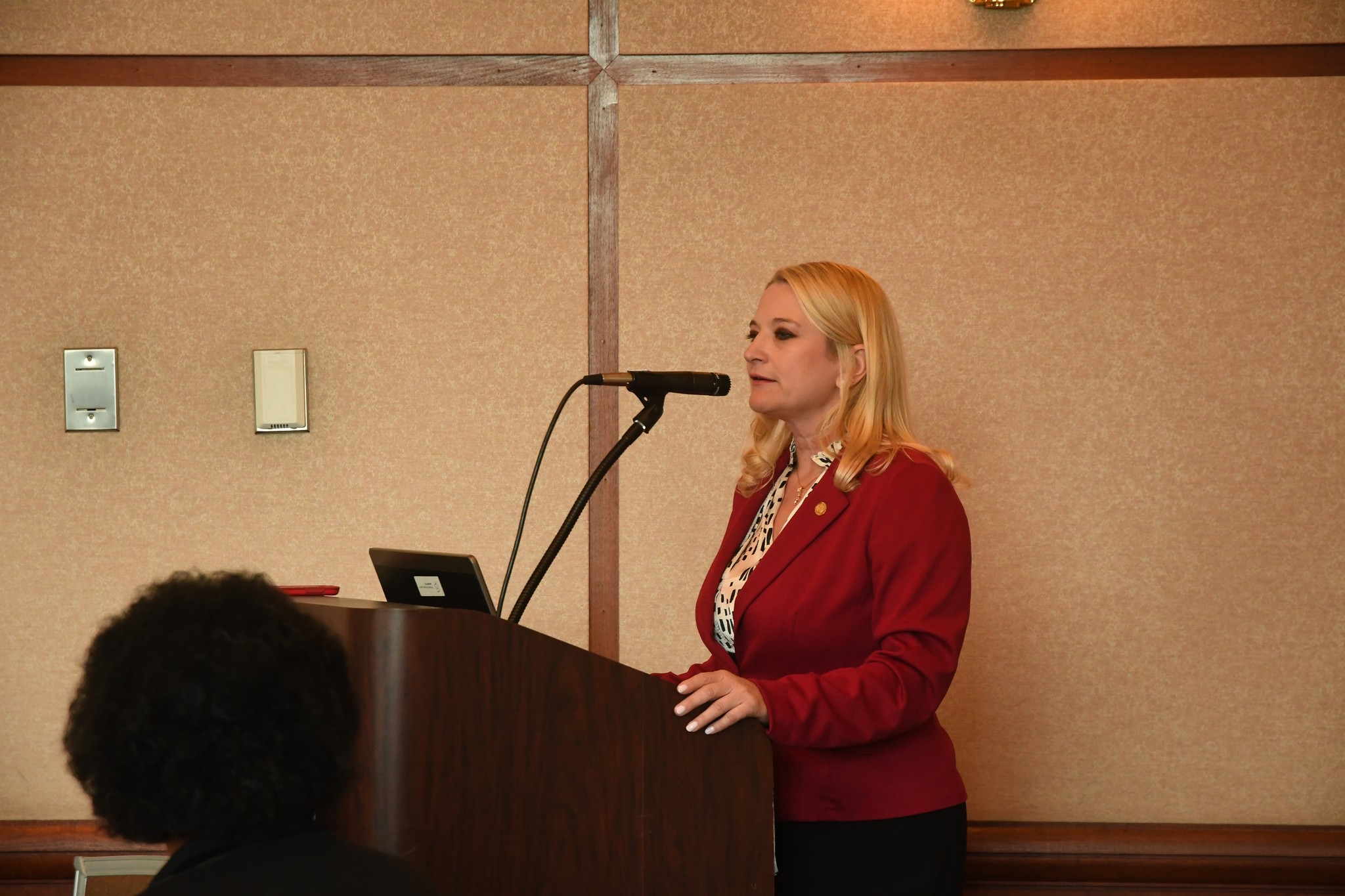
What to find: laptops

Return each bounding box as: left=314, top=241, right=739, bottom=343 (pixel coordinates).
left=368, top=547, right=497, bottom=616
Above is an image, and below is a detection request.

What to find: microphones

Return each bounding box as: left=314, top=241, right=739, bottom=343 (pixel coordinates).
left=584, top=370, right=730, bottom=396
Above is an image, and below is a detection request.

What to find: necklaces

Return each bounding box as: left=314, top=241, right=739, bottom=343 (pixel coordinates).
left=794, top=465, right=820, bottom=506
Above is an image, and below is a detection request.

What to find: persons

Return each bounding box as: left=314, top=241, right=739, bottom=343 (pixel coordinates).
left=61, top=570, right=439, bottom=896
left=646, top=260, right=972, bottom=895
